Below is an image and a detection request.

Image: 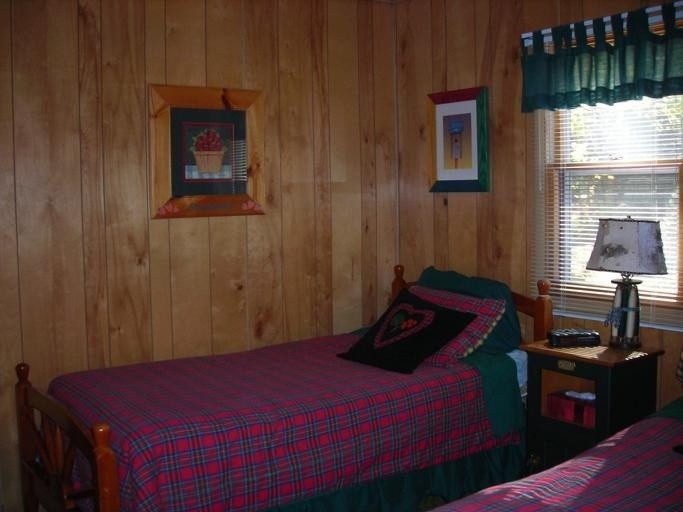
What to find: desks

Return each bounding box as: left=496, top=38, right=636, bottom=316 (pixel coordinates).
left=520, top=338, right=665, bottom=473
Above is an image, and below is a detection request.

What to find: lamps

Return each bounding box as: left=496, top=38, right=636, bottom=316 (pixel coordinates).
left=585, top=216, right=668, bottom=350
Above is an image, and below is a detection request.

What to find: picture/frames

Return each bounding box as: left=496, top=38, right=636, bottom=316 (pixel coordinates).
left=147, top=82, right=267, bottom=218
left=425, top=87, right=490, bottom=193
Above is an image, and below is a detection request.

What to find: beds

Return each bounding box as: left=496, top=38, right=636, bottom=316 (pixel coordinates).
left=425, top=394, right=683, bottom=512
left=14, top=261, right=552, bottom=512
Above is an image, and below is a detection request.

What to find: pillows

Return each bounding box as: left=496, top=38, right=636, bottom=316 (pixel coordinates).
left=335, top=263, right=522, bottom=374
